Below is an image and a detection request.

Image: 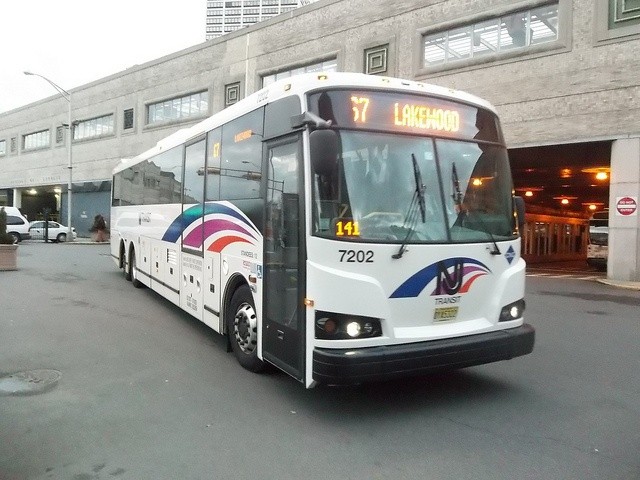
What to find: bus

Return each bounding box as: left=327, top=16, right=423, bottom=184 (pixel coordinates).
left=108, top=69, right=534, bottom=391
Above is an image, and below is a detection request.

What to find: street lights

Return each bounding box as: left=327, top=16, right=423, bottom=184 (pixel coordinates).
left=22, top=70, right=74, bottom=243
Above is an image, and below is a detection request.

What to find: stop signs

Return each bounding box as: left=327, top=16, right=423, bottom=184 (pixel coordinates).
left=616, top=197, right=637, bottom=216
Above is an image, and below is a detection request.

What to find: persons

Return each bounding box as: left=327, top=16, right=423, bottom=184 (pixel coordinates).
left=95, top=215, right=106, bottom=243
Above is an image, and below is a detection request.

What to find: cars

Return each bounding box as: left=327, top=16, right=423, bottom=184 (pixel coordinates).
left=21, top=220, right=77, bottom=243
left=1, top=213, right=30, bottom=244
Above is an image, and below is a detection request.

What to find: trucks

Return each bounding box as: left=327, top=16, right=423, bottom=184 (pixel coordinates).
left=585, top=207, right=609, bottom=272
